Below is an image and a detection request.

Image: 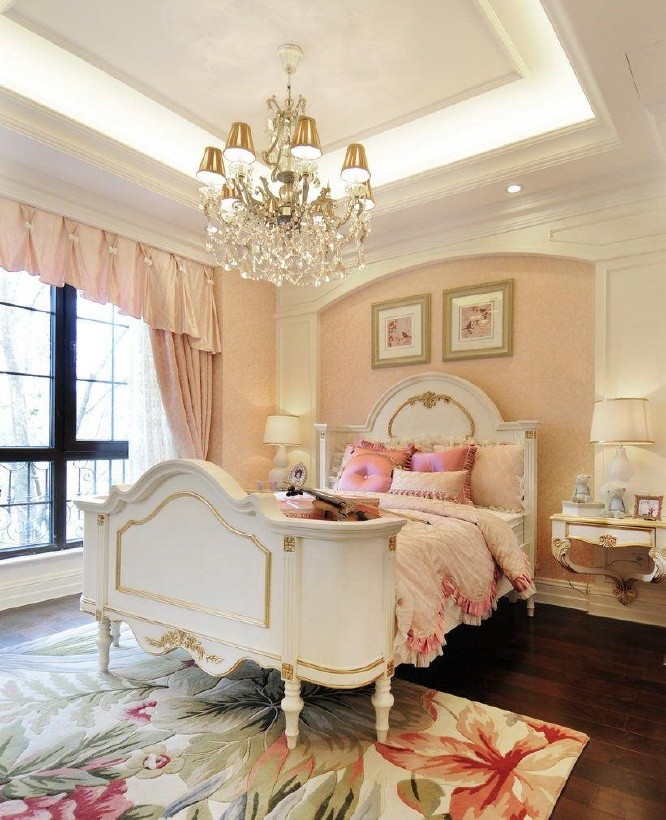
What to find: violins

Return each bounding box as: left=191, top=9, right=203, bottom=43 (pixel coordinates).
left=288, top=482, right=382, bottom=521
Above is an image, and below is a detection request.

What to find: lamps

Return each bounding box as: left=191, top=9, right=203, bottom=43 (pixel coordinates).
left=261, top=414, right=304, bottom=490
left=589, top=398, right=655, bottom=518
left=193, top=42, right=376, bottom=286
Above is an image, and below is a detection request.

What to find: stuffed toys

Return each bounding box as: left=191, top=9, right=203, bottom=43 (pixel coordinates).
left=571, top=474, right=592, bottom=503
left=601, top=486, right=627, bottom=519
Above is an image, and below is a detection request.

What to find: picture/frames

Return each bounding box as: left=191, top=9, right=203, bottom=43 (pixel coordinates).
left=442, top=278, right=514, bottom=361
left=632, top=494, right=664, bottom=521
left=370, top=292, right=431, bottom=368
left=286, top=461, right=308, bottom=486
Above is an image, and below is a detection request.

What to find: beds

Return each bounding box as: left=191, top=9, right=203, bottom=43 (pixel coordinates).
left=72, top=372, right=540, bottom=752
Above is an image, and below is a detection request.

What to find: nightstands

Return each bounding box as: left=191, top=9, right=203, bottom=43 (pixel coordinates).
left=550, top=511, right=666, bottom=606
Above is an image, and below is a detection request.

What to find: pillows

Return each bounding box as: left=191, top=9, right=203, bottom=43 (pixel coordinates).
left=332, top=440, right=415, bottom=496
left=385, top=466, right=470, bottom=505
left=410, top=444, right=478, bottom=504
left=433, top=438, right=526, bottom=514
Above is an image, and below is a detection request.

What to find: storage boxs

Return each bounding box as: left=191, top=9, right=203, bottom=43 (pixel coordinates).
left=562, top=501, right=606, bottom=518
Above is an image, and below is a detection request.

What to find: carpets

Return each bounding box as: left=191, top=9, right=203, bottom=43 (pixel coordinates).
left=0, top=620, right=592, bottom=820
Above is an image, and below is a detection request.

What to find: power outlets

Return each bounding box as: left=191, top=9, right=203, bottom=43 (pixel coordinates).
left=632, top=554, right=650, bottom=571
